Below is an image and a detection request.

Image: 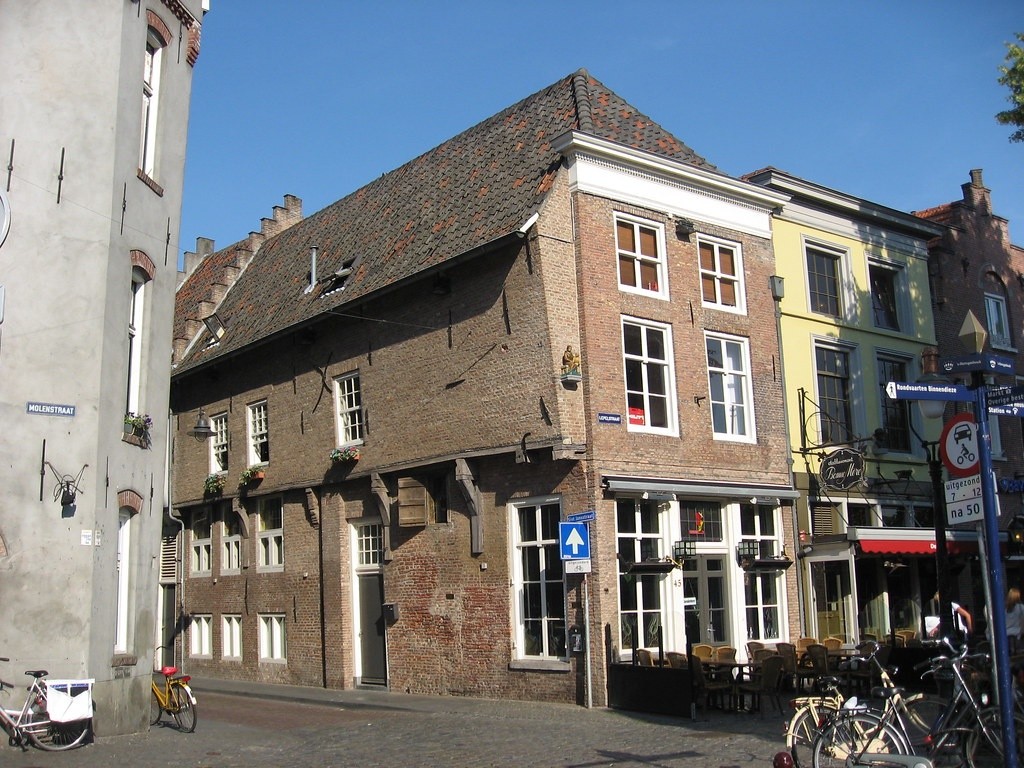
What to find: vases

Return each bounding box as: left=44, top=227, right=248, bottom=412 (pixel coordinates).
left=354, top=453, right=359, bottom=460
left=124, top=424, right=142, bottom=436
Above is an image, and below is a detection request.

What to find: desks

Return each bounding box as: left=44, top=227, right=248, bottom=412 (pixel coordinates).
left=653, top=659, right=763, bottom=715
left=796, top=648, right=862, bottom=699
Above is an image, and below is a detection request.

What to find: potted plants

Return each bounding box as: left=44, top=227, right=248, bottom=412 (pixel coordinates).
left=239, top=466, right=265, bottom=491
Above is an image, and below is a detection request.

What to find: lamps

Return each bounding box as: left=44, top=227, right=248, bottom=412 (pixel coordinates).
left=187, top=404, right=218, bottom=442
left=738, top=539, right=759, bottom=566
left=675, top=538, right=697, bottom=563
left=873, top=469, right=913, bottom=486
left=796, top=547, right=813, bottom=559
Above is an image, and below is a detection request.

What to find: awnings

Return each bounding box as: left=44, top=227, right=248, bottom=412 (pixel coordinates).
left=858, top=541, right=1018, bottom=556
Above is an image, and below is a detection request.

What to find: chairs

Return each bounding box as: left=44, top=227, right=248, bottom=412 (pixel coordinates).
left=639, top=630, right=917, bottom=720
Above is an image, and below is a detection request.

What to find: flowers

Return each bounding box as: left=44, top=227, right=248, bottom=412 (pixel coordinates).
left=329, top=446, right=359, bottom=462
left=125, top=412, right=153, bottom=442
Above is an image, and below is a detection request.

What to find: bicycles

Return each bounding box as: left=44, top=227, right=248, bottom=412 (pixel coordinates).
left=783, top=639, right=1024, bottom=767
left=150, top=646, right=197, bottom=733
left=0, top=657, right=95, bottom=751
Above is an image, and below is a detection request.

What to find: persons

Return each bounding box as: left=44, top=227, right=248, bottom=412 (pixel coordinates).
left=929, top=590, right=973, bottom=641
left=984, top=587, right=1024, bottom=686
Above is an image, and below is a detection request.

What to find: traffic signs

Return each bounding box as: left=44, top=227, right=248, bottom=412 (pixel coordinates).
left=986, top=384, right=1024, bottom=417
left=884, top=382, right=971, bottom=403
